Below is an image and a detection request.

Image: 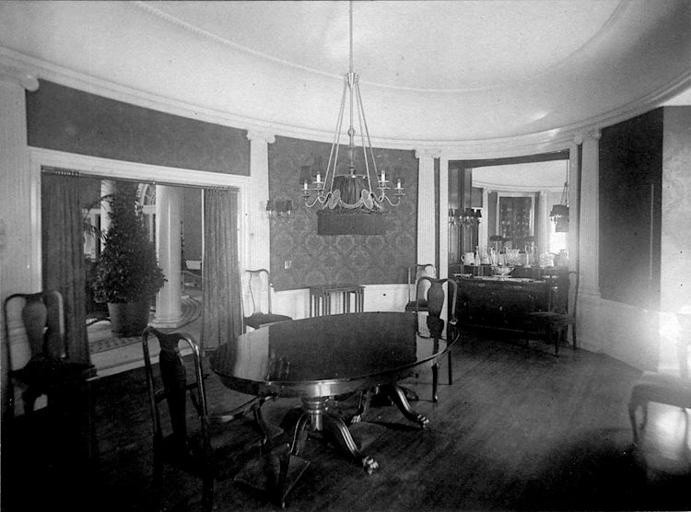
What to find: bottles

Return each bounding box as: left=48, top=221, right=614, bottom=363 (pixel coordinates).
left=474, top=245, right=481, bottom=266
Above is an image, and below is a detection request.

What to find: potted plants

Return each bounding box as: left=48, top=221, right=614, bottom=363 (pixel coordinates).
left=88, top=186, right=169, bottom=336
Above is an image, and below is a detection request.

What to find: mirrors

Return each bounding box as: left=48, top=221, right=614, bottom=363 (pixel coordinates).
left=459, top=151, right=570, bottom=271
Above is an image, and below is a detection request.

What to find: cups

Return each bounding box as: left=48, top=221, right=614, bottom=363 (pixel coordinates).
left=461, top=252, right=475, bottom=266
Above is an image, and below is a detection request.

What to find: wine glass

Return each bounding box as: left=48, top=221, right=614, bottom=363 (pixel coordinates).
left=491, top=267, right=514, bottom=282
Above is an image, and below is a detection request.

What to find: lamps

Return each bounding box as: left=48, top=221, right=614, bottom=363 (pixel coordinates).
left=474, top=207, right=482, bottom=226
left=454, top=207, right=473, bottom=226
left=303, top=0, right=404, bottom=210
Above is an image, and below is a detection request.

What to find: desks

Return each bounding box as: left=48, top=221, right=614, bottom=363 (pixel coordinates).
left=451, top=275, right=559, bottom=346
left=210, top=311, right=459, bottom=477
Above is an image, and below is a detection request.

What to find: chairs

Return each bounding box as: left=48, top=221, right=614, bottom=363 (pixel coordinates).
left=626, top=313, right=691, bottom=450
left=523, top=272, right=571, bottom=357
left=404, top=264, right=437, bottom=315
left=240, top=269, right=293, bottom=333
left=4, top=289, right=101, bottom=467
left=135, top=324, right=291, bottom=512
left=310, top=283, right=365, bottom=318
left=413, top=277, right=458, bottom=403
